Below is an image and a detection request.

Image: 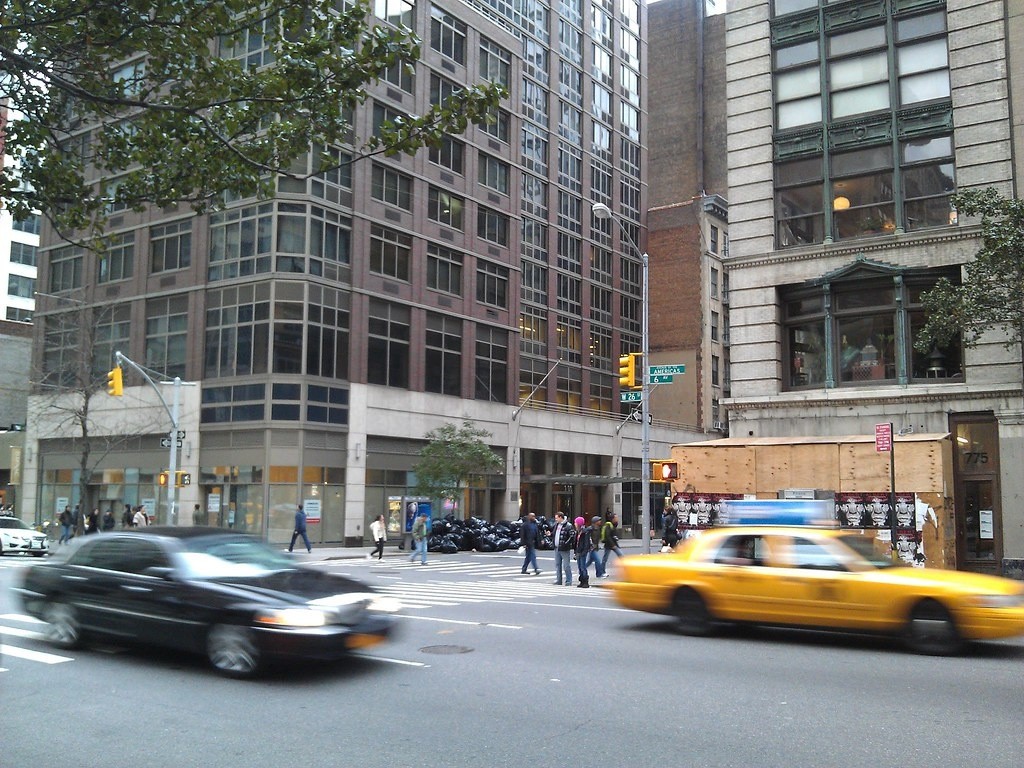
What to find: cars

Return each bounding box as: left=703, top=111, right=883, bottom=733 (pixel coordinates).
left=0, top=516, right=50, bottom=558
left=608, top=526, right=1024, bottom=656
left=18, top=526, right=404, bottom=677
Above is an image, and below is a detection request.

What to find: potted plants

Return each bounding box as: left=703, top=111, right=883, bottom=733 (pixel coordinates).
left=857, top=207, right=882, bottom=237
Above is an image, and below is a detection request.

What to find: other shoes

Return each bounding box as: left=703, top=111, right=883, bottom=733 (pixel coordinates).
left=565, top=583, right=571, bottom=586
left=284, top=549, right=292, bottom=553
left=377, top=558, right=386, bottom=563
left=582, top=580, right=589, bottom=588
left=536, top=571, right=540, bottom=575
left=577, top=580, right=582, bottom=587
left=596, top=574, right=609, bottom=579
left=554, top=582, right=562, bottom=585
left=410, top=557, right=413, bottom=562
left=368, top=553, right=373, bottom=559
left=521, top=572, right=530, bottom=574
left=308, top=550, right=311, bottom=553
left=422, top=562, right=427, bottom=565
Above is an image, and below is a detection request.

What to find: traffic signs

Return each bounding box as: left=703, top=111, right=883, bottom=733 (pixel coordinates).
left=876, top=424, right=891, bottom=452
left=161, top=438, right=182, bottom=448
left=630, top=408, right=653, bottom=425
left=166, top=430, right=186, bottom=438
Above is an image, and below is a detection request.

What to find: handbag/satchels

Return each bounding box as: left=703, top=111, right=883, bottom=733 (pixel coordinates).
left=676, top=533, right=683, bottom=541
left=662, top=546, right=674, bottom=553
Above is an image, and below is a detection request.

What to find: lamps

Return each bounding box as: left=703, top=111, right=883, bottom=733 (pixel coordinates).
left=834, top=184, right=850, bottom=209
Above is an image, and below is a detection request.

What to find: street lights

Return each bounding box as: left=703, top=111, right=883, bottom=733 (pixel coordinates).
left=591, top=202, right=651, bottom=555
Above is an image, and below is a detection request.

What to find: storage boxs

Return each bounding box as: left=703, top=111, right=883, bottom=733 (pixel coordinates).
left=801, top=299, right=822, bottom=309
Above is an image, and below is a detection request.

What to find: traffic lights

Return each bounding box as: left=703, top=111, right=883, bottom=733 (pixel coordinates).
left=108, top=368, right=123, bottom=396
left=177, top=472, right=190, bottom=486
left=618, top=356, right=635, bottom=387
left=653, top=462, right=681, bottom=481
left=160, top=472, right=168, bottom=486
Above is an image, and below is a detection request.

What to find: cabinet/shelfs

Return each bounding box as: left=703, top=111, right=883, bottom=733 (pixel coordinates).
left=790, top=327, right=816, bottom=386
left=782, top=196, right=813, bottom=243
left=851, top=360, right=885, bottom=382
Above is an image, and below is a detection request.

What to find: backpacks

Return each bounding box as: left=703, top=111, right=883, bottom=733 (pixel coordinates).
left=601, top=522, right=613, bottom=543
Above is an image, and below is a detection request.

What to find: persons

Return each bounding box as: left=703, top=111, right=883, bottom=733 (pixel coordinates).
left=283, top=504, right=311, bottom=553
left=0, top=504, right=13, bottom=516
left=73, top=505, right=82, bottom=535
left=120, top=504, right=150, bottom=527
left=573, top=517, right=590, bottom=588
left=366, top=515, right=387, bottom=562
left=85, top=507, right=101, bottom=535
left=661, top=505, right=678, bottom=548
left=408, top=513, right=428, bottom=565
left=545, top=511, right=575, bottom=586
left=586, top=516, right=606, bottom=579
left=601, top=514, right=624, bottom=577
left=103, top=509, right=115, bottom=531
left=58, top=505, right=75, bottom=545
left=605, top=507, right=611, bottom=519
left=192, top=504, right=205, bottom=525
left=521, top=513, right=542, bottom=575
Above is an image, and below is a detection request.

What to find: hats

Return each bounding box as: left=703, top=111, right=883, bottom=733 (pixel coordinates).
left=574, top=517, right=585, bottom=526
left=591, top=516, right=601, bottom=522
